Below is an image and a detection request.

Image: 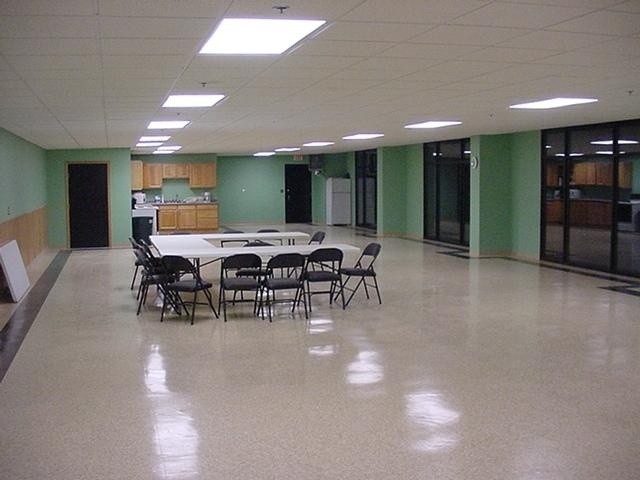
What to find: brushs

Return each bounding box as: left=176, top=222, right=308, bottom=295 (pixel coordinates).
left=203, top=192, right=211, bottom=203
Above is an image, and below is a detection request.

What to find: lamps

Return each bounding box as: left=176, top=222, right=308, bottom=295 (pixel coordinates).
left=127, top=229, right=383, bottom=326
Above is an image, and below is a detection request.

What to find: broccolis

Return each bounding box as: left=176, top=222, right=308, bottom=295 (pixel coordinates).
left=325, top=177, right=351, bottom=225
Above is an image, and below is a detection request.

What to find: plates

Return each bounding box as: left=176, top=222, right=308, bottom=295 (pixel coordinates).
left=157, top=205, right=197, bottom=230
left=189, top=162, right=217, bottom=189
left=143, top=163, right=163, bottom=189
left=163, top=163, right=190, bottom=179
left=546, top=199, right=615, bottom=226
left=546, top=161, right=633, bottom=188
left=131, top=160, right=144, bottom=191
left=197, top=205, right=219, bottom=230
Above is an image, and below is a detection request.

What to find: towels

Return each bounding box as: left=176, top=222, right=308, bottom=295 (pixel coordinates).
left=133, top=216, right=153, bottom=246
